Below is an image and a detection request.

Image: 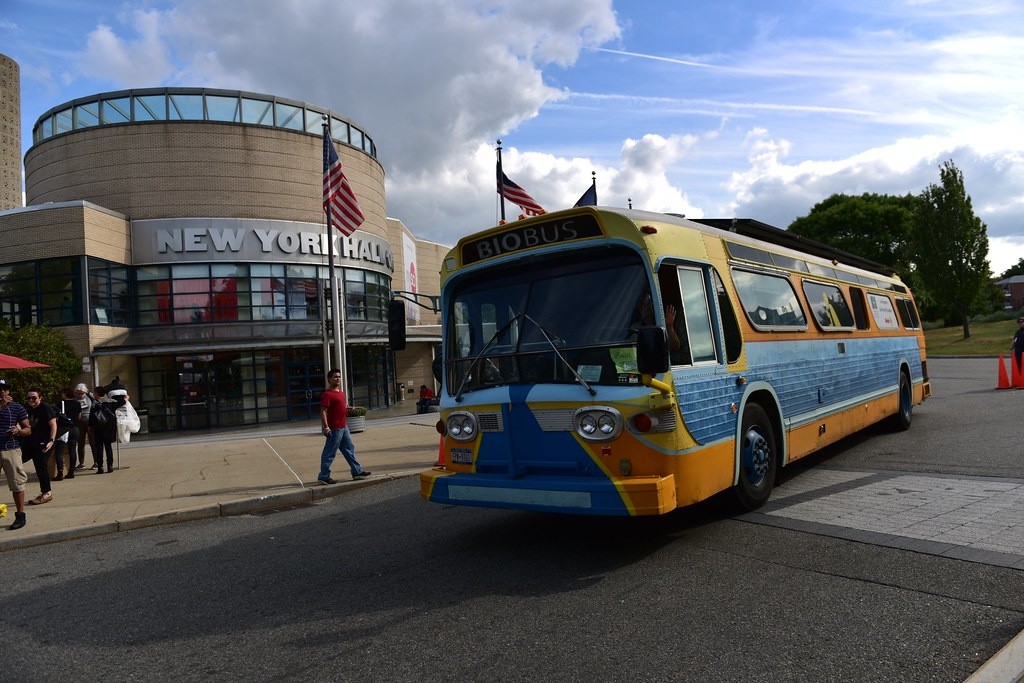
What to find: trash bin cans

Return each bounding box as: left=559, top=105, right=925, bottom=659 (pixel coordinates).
left=136, top=409, right=149, bottom=435
left=396, top=383, right=405, bottom=401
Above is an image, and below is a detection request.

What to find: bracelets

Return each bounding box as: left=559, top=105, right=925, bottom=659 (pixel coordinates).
left=323, top=426, right=327, bottom=429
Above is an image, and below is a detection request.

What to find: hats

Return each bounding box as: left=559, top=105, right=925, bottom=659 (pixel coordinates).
left=0, top=380, right=11, bottom=388
left=75, top=383, right=89, bottom=393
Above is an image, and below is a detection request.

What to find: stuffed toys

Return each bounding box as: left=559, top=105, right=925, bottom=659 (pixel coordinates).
left=101, top=375, right=141, bottom=446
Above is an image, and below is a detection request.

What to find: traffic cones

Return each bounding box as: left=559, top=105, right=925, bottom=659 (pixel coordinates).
left=1008, top=350, right=1022, bottom=388
left=1018, top=351, right=1024, bottom=388
left=995, top=353, right=1013, bottom=389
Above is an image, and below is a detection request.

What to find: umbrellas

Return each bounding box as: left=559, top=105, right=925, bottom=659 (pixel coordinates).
left=0, top=353, right=53, bottom=370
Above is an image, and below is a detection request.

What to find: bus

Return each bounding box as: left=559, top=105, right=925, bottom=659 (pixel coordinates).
left=420, top=208, right=933, bottom=518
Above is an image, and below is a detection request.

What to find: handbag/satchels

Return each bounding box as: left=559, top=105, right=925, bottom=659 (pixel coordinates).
left=94, top=401, right=107, bottom=425
left=52, top=405, right=73, bottom=438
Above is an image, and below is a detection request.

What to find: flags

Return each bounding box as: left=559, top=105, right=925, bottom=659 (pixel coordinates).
left=496, top=148, right=546, bottom=216
left=321, top=122, right=365, bottom=238
left=571, top=183, right=598, bottom=208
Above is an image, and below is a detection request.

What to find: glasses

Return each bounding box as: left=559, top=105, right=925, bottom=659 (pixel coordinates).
left=26, top=396, right=37, bottom=401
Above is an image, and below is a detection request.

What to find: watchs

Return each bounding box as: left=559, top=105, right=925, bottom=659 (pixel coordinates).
left=49, top=438, right=55, bottom=444
left=17, top=428, right=23, bottom=437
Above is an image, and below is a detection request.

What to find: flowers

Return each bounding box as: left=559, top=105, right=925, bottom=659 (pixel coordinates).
left=346, top=406, right=367, bottom=417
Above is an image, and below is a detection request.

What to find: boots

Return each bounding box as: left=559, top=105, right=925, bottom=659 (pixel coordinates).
left=51, top=471, right=63, bottom=481
left=64, top=472, right=74, bottom=479
left=9, top=512, right=26, bottom=530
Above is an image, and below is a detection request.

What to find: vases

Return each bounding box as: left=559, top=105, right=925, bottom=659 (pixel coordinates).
left=347, top=416, right=366, bottom=432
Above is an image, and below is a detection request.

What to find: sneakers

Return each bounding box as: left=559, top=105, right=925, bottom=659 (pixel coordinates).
left=353, top=472, right=372, bottom=480
left=317, top=477, right=337, bottom=485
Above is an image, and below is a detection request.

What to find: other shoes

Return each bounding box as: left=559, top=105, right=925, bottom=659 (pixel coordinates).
left=108, top=469, right=113, bottom=473
left=28, top=493, right=52, bottom=505
left=75, top=464, right=85, bottom=470
left=96, top=469, right=104, bottom=474
left=91, top=464, right=99, bottom=470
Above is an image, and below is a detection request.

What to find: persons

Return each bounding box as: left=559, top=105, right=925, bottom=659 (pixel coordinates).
left=0, top=379, right=32, bottom=529
left=625, top=288, right=681, bottom=353
left=17, top=387, right=58, bottom=506
left=1009, top=316, right=1024, bottom=373
left=318, top=368, right=371, bottom=485
left=52, top=381, right=130, bottom=481
left=418, top=385, right=432, bottom=414
